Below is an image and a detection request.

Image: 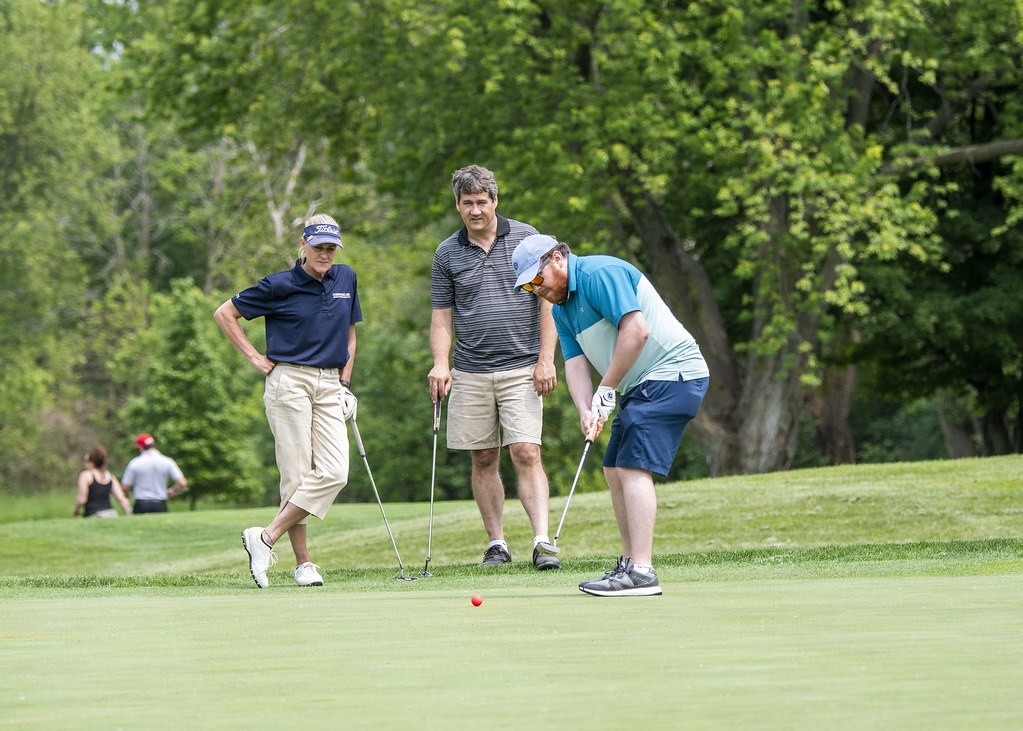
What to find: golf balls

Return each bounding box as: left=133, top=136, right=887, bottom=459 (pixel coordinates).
left=471, top=594, right=483, bottom=606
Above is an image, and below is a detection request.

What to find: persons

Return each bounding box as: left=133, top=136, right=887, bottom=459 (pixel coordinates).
left=427, top=164, right=558, bottom=571
left=213, top=214, right=363, bottom=588
left=71, top=446, right=132, bottom=519
left=511, top=234, right=710, bottom=596
left=121, top=433, right=187, bottom=515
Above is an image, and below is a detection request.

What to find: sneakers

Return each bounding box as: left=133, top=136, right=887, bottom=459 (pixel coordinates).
left=532, top=541, right=559, bottom=570
left=241, top=527, right=279, bottom=588
left=481, top=544, right=512, bottom=567
left=578, top=554, right=663, bottom=597
left=294, top=562, right=323, bottom=587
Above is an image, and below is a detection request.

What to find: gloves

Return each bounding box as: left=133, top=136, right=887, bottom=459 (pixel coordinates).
left=591, top=385, right=616, bottom=423
left=341, top=386, right=357, bottom=422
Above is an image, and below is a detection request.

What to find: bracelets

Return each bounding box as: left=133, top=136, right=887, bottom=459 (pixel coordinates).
left=339, top=380, right=351, bottom=388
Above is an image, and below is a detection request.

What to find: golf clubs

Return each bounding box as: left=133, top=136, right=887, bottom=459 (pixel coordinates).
left=349, top=413, right=419, bottom=582
left=421, top=397, right=442, bottom=578
left=537, top=412, right=602, bottom=556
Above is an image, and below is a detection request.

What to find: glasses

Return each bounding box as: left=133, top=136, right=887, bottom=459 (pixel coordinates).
left=521, top=256, right=550, bottom=292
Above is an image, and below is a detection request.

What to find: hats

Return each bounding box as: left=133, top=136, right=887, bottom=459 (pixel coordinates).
left=304, top=224, right=345, bottom=250
left=511, top=234, right=559, bottom=294
left=136, top=433, right=154, bottom=448
